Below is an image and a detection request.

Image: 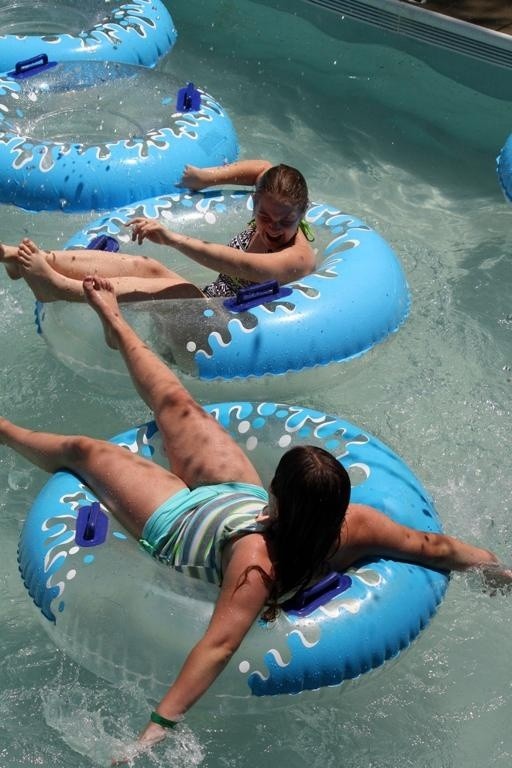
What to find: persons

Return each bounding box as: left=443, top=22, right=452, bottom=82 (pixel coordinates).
left=1, top=275, right=509, bottom=764
left=1, top=157, right=316, bottom=378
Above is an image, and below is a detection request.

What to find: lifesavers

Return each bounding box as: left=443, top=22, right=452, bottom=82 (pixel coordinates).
left=0, top=55, right=240, bottom=212
left=1, top=1, right=179, bottom=92
left=36, top=186, right=411, bottom=403
left=17, top=402, right=447, bottom=719
left=496, top=130, right=511, bottom=210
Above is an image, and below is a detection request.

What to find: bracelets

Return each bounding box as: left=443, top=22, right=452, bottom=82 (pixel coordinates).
left=151, top=711, right=176, bottom=729
